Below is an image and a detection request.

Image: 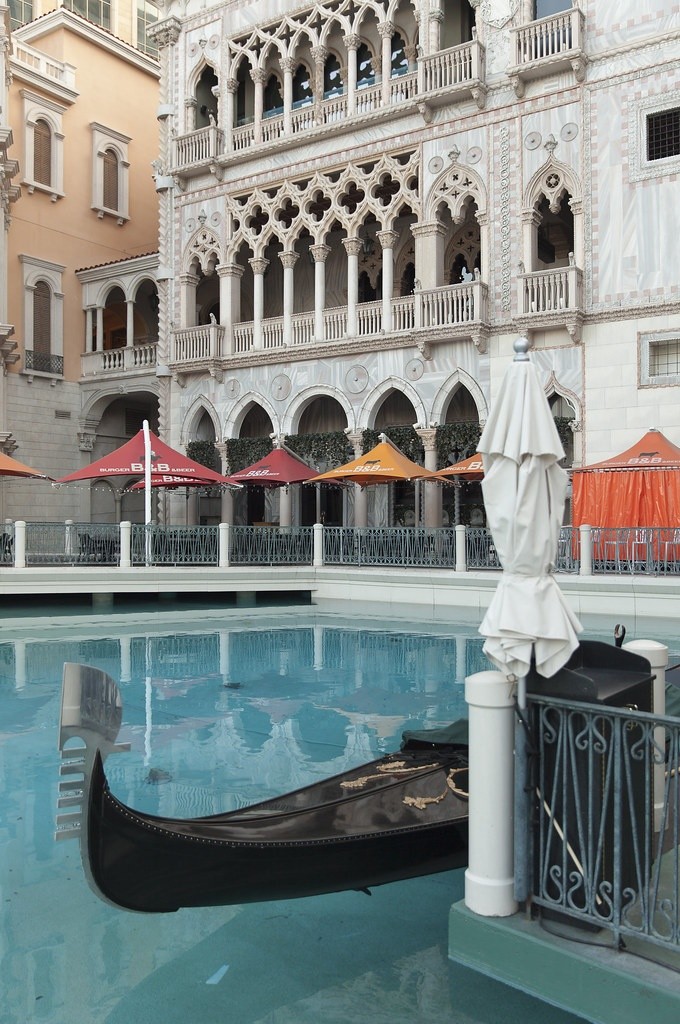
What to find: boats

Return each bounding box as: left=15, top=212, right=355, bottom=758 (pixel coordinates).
left=57, top=626, right=680, bottom=915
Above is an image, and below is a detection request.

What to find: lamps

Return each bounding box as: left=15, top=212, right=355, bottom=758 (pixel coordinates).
left=344, top=428, right=352, bottom=434
left=429, top=422, right=437, bottom=428
left=413, top=422, right=421, bottom=429
left=268, top=433, right=276, bottom=439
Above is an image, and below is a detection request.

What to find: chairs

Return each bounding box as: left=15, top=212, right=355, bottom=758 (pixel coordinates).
left=0, top=530, right=498, bottom=569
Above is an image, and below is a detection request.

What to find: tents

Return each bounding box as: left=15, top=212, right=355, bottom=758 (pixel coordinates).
left=570, top=427, right=680, bottom=562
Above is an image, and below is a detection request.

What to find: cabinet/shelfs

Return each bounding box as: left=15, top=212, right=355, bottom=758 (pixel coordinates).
left=524, top=639, right=657, bottom=933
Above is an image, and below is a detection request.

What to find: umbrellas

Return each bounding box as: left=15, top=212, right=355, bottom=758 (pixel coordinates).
left=480, top=337, right=583, bottom=905
left=422, top=450, right=486, bottom=478
left=303, top=434, right=462, bottom=527
left=0, top=452, right=53, bottom=481
left=51, top=423, right=246, bottom=564
left=225, top=441, right=349, bottom=524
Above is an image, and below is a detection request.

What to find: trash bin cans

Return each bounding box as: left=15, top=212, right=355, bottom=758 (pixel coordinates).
left=526, top=636, right=658, bottom=930
